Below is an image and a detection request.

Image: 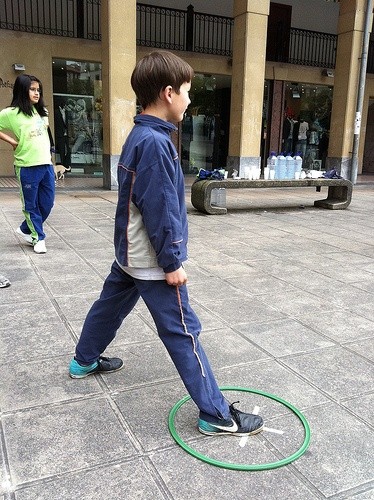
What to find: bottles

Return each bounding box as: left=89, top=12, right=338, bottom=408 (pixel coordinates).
left=267, top=151, right=302, bottom=180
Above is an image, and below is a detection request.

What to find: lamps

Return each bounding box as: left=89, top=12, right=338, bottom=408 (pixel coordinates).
left=12, top=63, right=26, bottom=71
left=321, top=70, right=334, bottom=78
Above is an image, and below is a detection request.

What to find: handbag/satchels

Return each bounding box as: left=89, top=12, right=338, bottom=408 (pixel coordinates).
left=199, top=169, right=224, bottom=180
left=322, top=167, right=343, bottom=179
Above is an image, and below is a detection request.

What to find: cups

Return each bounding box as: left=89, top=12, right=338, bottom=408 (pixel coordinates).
left=264, top=167, right=274, bottom=180
left=295, top=172, right=300, bottom=180
left=244, top=166, right=261, bottom=180
left=214, top=169, right=228, bottom=180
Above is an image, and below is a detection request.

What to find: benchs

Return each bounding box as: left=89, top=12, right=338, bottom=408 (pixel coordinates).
left=191, top=177, right=353, bottom=214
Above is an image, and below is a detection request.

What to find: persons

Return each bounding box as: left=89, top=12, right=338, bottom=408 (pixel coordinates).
left=283, top=114, right=323, bottom=165
left=0, top=73, right=56, bottom=252
left=70, top=51, right=266, bottom=437
left=58, top=99, right=103, bottom=153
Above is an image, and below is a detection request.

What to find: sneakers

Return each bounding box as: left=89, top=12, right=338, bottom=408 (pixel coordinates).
left=69, top=355, right=124, bottom=379
left=0, top=274, right=11, bottom=287
left=16, top=227, right=32, bottom=244
left=199, top=400, right=264, bottom=436
left=34, top=240, right=46, bottom=253
